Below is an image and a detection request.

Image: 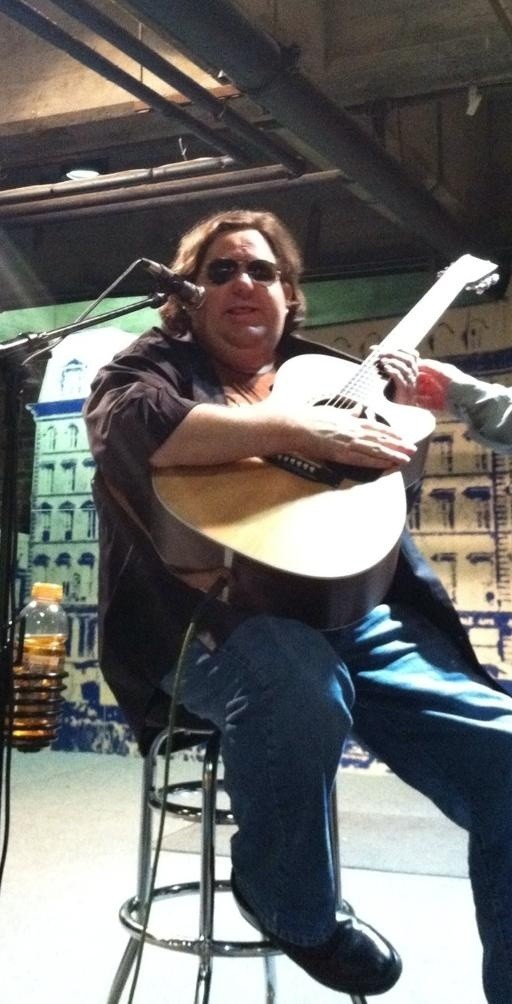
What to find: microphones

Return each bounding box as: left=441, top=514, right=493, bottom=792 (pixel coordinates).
left=142, top=260, right=206, bottom=311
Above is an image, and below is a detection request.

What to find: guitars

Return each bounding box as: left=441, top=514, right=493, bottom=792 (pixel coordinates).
left=139, top=250, right=500, bottom=633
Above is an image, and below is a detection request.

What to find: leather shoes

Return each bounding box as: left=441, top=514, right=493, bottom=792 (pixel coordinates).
left=229, top=866, right=404, bottom=996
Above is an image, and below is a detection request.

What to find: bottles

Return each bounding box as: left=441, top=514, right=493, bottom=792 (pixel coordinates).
left=9, top=582, right=70, bottom=736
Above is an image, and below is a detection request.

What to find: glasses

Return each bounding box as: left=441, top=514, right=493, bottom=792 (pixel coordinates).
left=196, top=257, right=285, bottom=286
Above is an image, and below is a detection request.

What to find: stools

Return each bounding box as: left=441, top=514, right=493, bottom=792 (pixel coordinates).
left=105, top=720, right=369, bottom=1004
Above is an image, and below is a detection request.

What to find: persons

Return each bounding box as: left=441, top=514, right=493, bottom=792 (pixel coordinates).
left=413, top=358, right=511, bottom=455
left=85, top=210, right=511, bottom=1002
